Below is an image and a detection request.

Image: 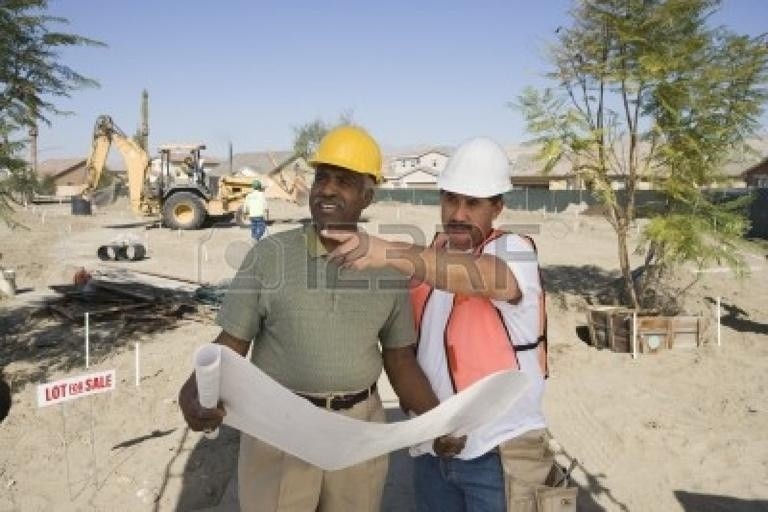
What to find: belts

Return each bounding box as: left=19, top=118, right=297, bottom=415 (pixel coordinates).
left=294, top=380, right=378, bottom=411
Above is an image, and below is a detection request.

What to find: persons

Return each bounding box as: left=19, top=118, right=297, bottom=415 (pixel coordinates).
left=318, top=135, right=551, bottom=511
left=242, top=178, right=270, bottom=245
left=177, top=120, right=467, bottom=512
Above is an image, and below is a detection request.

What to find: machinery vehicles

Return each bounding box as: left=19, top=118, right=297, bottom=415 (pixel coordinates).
left=72, top=116, right=269, bottom=229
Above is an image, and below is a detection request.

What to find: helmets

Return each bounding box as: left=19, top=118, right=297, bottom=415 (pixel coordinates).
left=309, top=125, right=384, bottom=186
left=251, top=180, right=262, bottom=189
left=437, top=136, right=515, bottom=200
